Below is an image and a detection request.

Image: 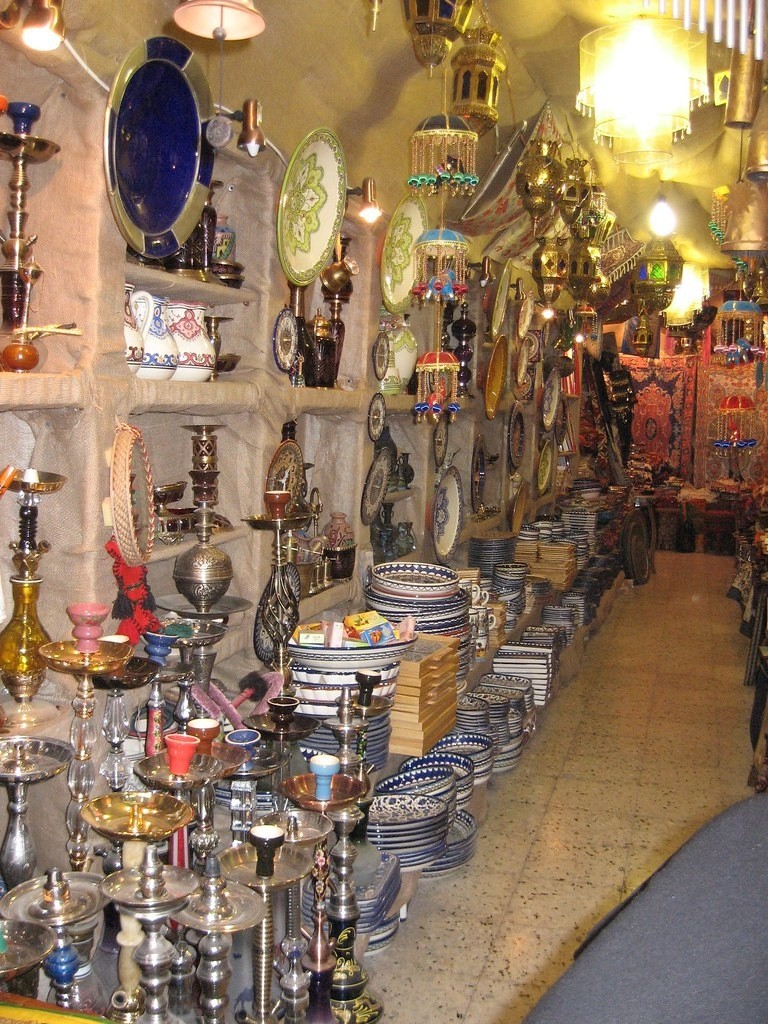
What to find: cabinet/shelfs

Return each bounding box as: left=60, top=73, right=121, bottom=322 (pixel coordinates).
left=0, top=1, right=583, bottom=742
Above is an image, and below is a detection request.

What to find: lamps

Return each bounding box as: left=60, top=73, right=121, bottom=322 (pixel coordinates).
left=1, top=0, right=66, bottom=52
left=713, top=392, right=757, bottom=492
left=173, top=0, right=267, bottom=148
left=345, top=178, right=382, bottom=224
left=718, top=301, right=767, bottom=374
left=402, top=0, right=507, bottom=425
left=575, top=14, right=710, bottom=175
left=469, top=255, right=494, bottom=286
left=535, top=298, right=554, bottom=318
left=510, top=276, right=525, bottom=301
left=216, top=97, right=266, bottom=158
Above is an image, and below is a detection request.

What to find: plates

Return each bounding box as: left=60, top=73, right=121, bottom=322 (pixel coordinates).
left=105, top=35, right=682, bottom=958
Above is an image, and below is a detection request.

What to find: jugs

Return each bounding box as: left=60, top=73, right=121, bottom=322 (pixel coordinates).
left=166, top=302, right=215, bottom=381
left=133, top=295, right=179, bottom=381
left=120, top=282, right=153, bottom=372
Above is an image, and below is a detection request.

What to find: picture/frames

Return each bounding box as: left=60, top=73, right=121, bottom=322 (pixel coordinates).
left=388, top=633, right=460, bottom=756
left=516, top=540, right=578, bottom=592
left=479, top=599, right=508, bottom=648
left=454, top=566, right=481, bottom=587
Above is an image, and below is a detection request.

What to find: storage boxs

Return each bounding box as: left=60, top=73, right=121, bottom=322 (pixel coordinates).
left=290, top=609, right=417, bottom=649
